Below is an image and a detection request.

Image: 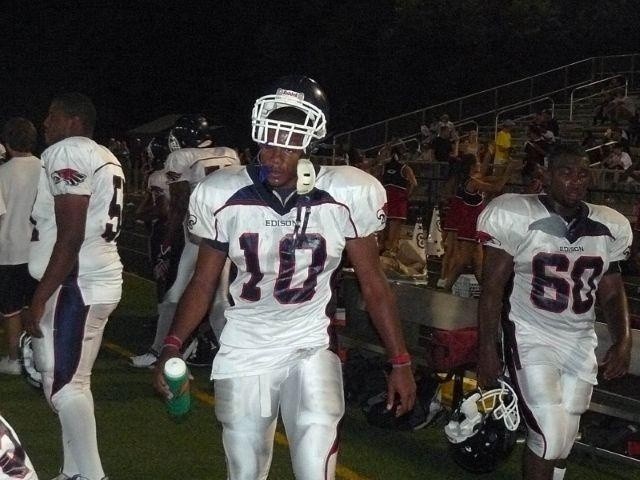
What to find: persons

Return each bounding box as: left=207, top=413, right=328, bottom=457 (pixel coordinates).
left=476, top=141, right=637, bottom=480
left=133, top=133, right=184, bottom=309
left=276, top=81, right=639, bottom=305
left=0, top=115, right=41, bottom=381
left=150, top=74, right=421, bottom=480
left=18, top=87, right=126, bottom=480
left=390, top=352, right=412, bottom=368
left=125, top=115, right=236, bottom=370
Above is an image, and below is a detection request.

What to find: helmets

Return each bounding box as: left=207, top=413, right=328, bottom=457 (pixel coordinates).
left=259, top=72, right=332, bottom=141
left=149, top=136, right=170, bottom=160
left=171, top=112, right=212, bottom=148
left=442, top=386, right=518, bottom=476
left=18, top=330, right=43, bottom=390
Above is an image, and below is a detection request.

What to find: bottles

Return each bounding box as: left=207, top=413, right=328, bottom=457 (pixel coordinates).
left=162, top=358, right=191, bottom=422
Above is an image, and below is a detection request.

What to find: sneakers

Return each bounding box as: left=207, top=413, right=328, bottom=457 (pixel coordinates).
left=185, top=347, right=220, bottom=368
left=0, top=355, right=23, bottom=376
left=128, top=350, right=160, bottom=370
left=50, top=472, right=110, bottom=480
